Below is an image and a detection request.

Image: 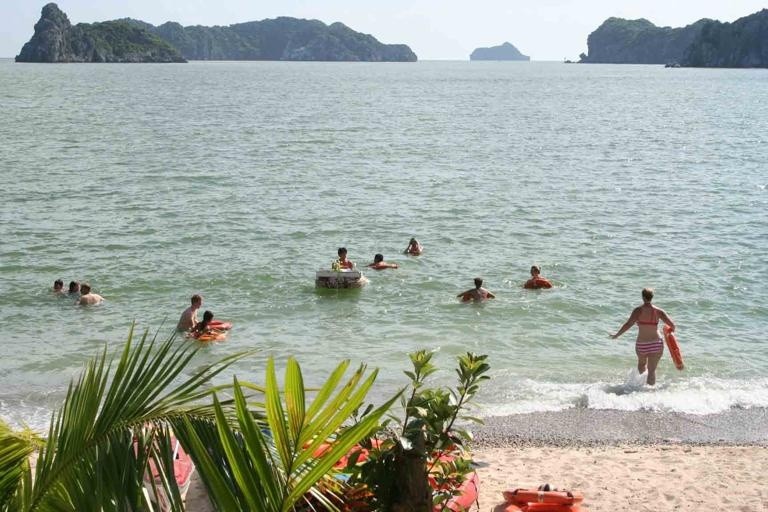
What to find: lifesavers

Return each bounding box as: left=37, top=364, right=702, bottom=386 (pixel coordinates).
left=190, top=331, right=225, bottom=342
left=492, top=483, right=586, bottom=512
left=200, top=321, right=231, bottom=329
left=463, top=294, right=492, bottom=302
left=525, top=279, right=551, bottom=288
left=663, top=325, right=684, bottom=369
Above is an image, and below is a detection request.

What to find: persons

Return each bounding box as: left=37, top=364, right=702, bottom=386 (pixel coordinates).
left=523, top=265, right=547, bottom=287
left=456, top=277, right=495, bottom=301
left=75, top=282, right=105, bottom=305
left=188, top=310, right=213, bottom=339
left=404, top=238, right=423, bottom=255
left=608, top=288, right=674, bottom=387
left=332, top=248, right=354, bottom=270
left=175, top=294, right=201, bottom=332
left=67, top=280, right=80, bottom=293
left=369, top=253, right=397, bottom=269
left=53, top=278, right=63, bottom=291
